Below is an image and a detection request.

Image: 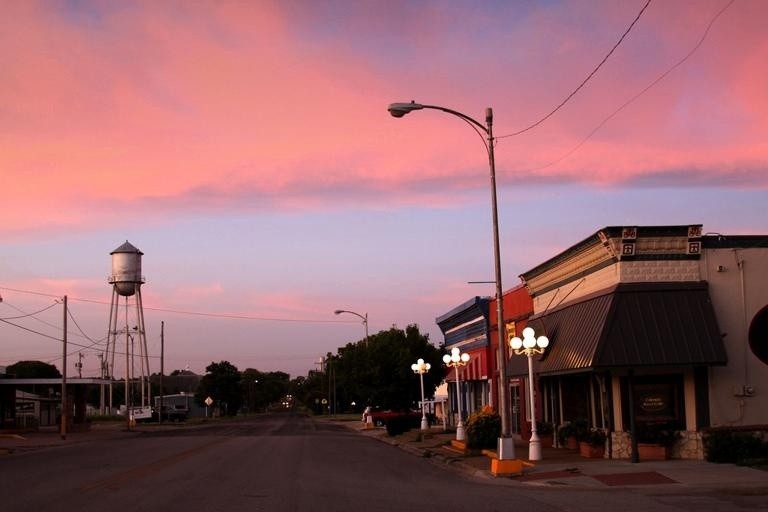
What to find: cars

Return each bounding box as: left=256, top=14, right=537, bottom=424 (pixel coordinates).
left=135, top=406, right=186, bottom=422
left=361, top=405, right=430, bottom=426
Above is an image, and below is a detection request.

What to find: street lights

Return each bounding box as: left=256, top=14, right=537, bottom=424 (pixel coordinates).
left=387, top=99, right=523, bottom=477
left=410, top=358, right=431, bottom=431
left=510, top=327, right=549, bottom=461
left=443, top=347, right=471, bottom=441
left=334, top=309, right=369, bottom=347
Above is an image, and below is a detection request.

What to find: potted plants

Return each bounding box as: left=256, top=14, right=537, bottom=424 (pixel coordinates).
left=637, top=432, right=680, bottom=461
left=557, top=425, right=606, bottom=459
